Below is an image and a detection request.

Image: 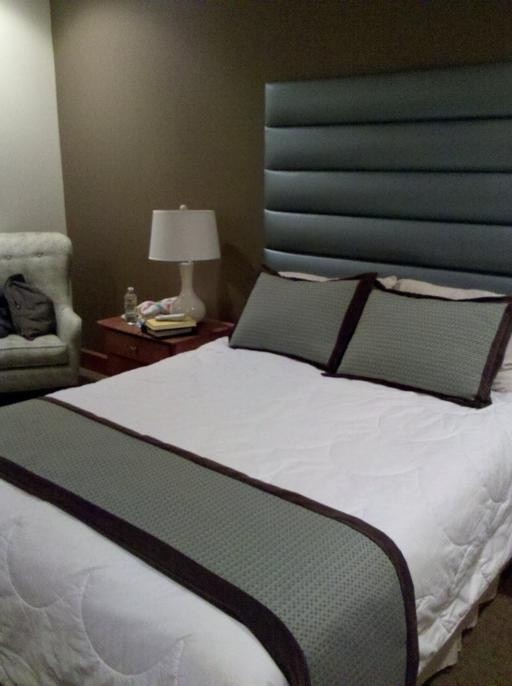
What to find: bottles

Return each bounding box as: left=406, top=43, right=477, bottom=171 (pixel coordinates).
left=123, top=286, right=137, bottom=325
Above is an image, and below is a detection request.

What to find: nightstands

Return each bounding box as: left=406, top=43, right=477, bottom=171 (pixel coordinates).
left=96, top=311, right=235, bottom=376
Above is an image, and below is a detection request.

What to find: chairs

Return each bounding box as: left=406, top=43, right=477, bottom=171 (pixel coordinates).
left=0, top=232, right=83, bottom=393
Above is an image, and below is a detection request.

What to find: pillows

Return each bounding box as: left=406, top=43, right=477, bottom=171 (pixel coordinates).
left=394, top=278, right=512, bottom=395
left=323, top=279, right=511, bottom=408
left=276, top=266, right=396, bottom=290
left=230, top=267, right=375, bottom=370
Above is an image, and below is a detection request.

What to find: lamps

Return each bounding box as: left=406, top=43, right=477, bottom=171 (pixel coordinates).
left=148, top=205, right=221, bottom=323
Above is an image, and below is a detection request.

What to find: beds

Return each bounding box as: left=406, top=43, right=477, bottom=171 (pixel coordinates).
left=0, top=61, right=512, bottom=685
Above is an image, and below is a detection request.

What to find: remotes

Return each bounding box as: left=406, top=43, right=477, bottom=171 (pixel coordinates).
left=155, top=313, right=185, bottom=321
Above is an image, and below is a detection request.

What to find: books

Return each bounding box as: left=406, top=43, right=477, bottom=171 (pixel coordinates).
left=141, top=315, right=196, bottom=338
